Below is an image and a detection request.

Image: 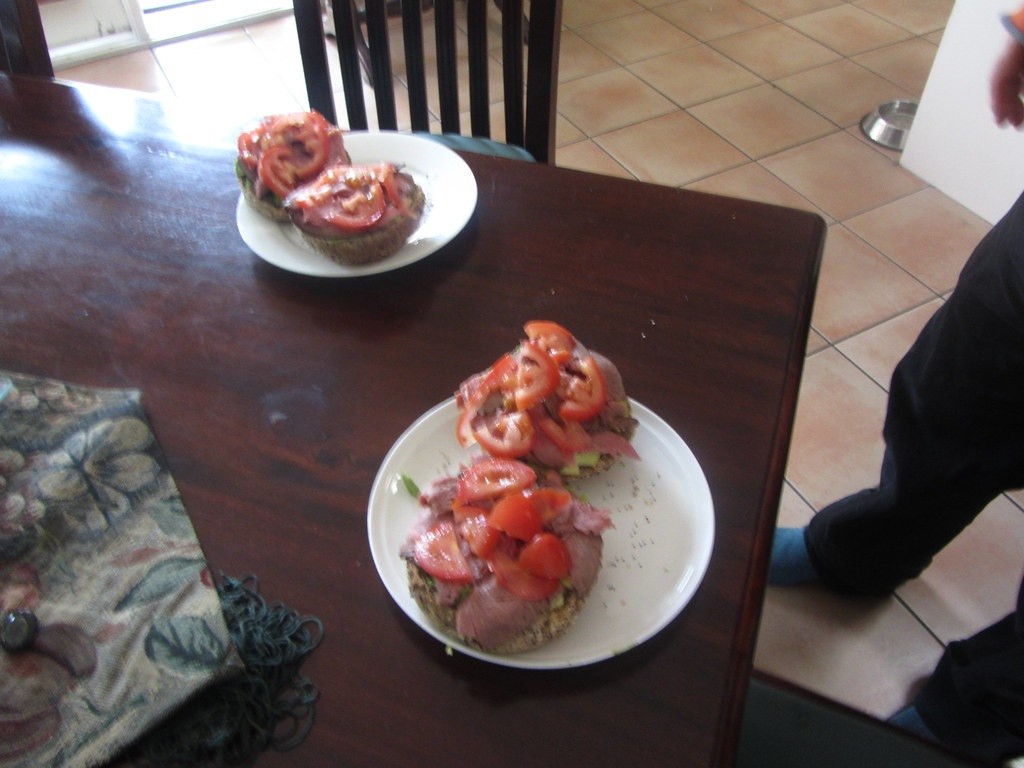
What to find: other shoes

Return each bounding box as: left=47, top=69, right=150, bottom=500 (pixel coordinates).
left=888, top=704, right=935, bottom=741
left=767, top=529, right=894, bottom=604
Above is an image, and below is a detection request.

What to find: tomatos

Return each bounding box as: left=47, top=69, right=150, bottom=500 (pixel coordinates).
left=413, top=456, right=573, bottom=600
left=237, top=108, right=411, bottom=233
left=456, top=320, right=607, bottom=458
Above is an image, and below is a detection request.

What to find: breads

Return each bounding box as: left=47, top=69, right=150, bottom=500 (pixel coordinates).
left=292, top=184, right=425, bottom=264
left=520, top=409, right=633, bottom=481
left=404, top=558, right=581, bottom=654
left=239, top=176, right=291, bottom=223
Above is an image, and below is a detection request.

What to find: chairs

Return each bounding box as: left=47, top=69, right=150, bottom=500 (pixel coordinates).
left=291, top=0, right=566, bottom=167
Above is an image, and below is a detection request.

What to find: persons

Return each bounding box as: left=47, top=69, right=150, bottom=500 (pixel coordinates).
left=763, top=0, right=1023, bottom=762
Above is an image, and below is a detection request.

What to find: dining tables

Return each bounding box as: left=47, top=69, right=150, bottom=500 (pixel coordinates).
left=0, top=75, right=827, bottom=767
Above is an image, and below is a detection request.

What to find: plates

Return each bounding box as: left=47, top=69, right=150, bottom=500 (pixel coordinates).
left=367, top=375, right=718, bottom=672
left=234, top=131, right=479, bottom=277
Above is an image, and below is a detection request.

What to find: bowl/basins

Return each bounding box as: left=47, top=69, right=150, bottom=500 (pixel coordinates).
left=860, top=99, right=920, bottom=153
